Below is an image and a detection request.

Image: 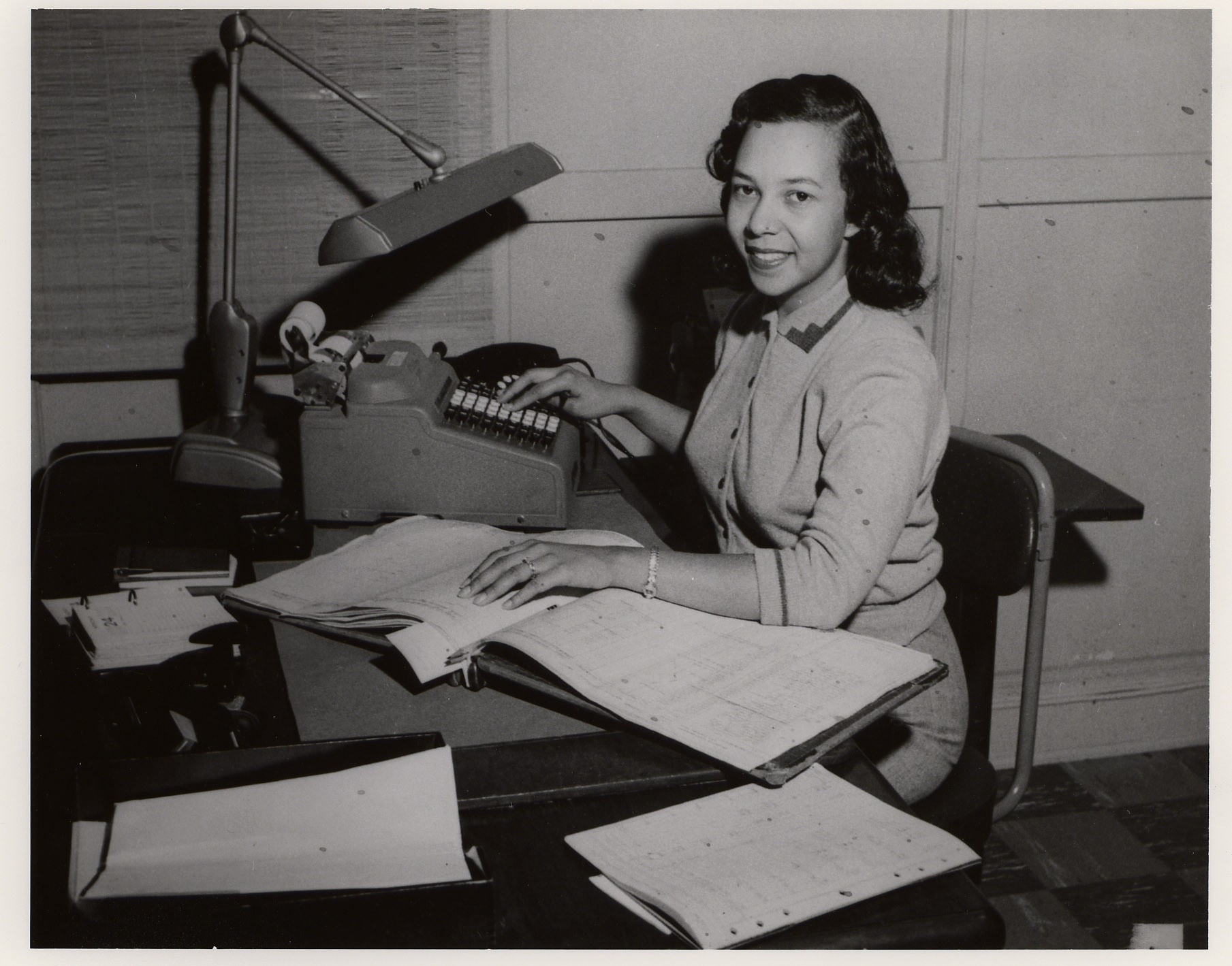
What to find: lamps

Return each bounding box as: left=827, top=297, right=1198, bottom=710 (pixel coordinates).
left=172, top=9, right=561, bottom=481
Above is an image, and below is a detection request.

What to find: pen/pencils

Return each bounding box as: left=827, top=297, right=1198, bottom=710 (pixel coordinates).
left=443, top=604, right=560, bottom=665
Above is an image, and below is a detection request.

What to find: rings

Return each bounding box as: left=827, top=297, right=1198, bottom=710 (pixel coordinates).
left=521, top=557, right=536, bottom=580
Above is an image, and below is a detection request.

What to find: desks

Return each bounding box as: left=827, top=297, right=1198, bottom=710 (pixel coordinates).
left=31, top=437, right=1009, bottom=950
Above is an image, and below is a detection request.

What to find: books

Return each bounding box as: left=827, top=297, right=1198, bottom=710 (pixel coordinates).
left=565, top=761, right=985, bottom=949
left=221, top=511, right=951, bottom=788
left=67, top=746, right=473, bottom=905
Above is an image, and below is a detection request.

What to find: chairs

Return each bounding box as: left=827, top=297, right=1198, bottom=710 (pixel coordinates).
left=936, top=437, right=1057, bottom=890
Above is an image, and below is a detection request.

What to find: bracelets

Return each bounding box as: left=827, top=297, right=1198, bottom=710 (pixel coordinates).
left=643, top=545, right=660, bottom=599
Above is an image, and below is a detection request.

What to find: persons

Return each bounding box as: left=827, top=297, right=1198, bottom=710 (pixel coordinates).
left=459, top=73, right=968, bottom=805
